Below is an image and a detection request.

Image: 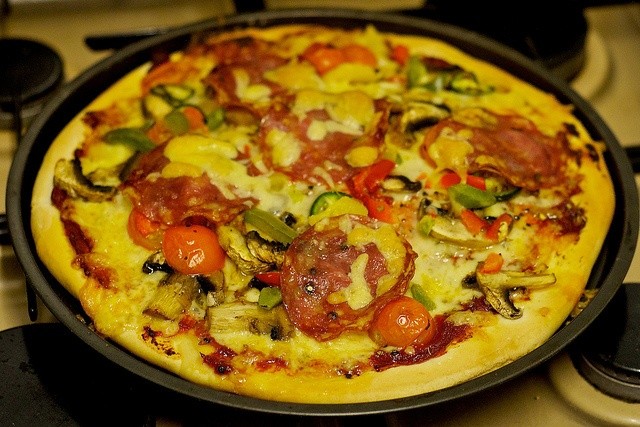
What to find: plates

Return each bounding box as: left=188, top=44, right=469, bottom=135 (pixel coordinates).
left=6, top=8, right=639, bottom=416
left=548, top=361, right=636, bottom=424
left=573, top=25, right=611, bottom=101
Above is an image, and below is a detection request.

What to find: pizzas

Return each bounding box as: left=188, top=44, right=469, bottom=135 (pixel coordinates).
left=28, top=25, right=615, bottom=404
